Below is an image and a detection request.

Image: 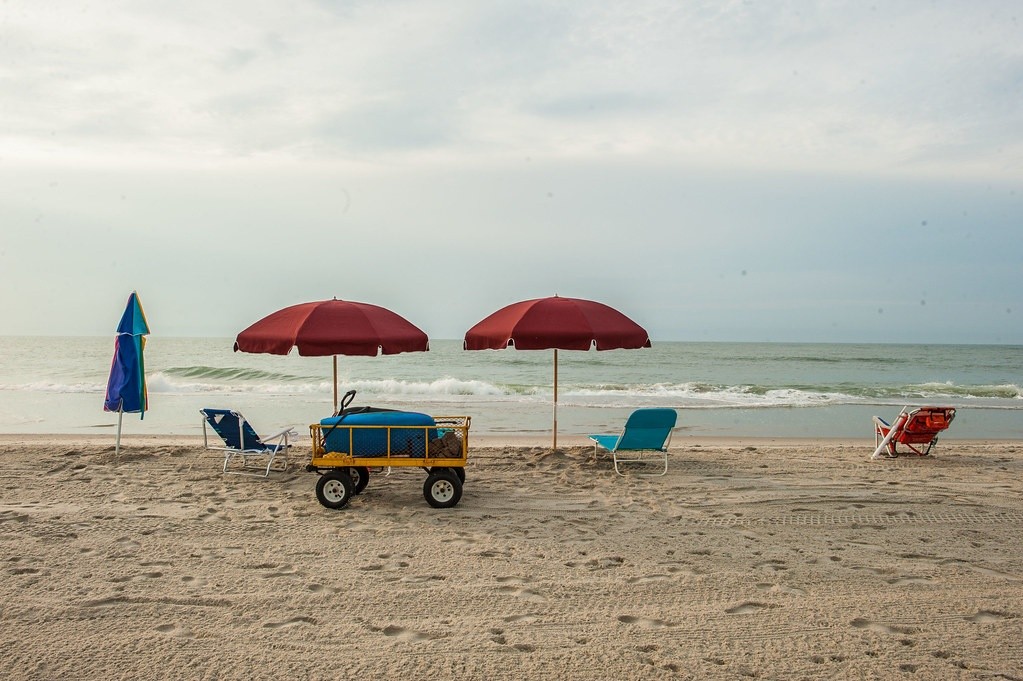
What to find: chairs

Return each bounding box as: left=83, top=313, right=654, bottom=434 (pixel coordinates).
left=870, top=405, right=957, bottom=461
left=586, top=406, right=678, bottom=477
left=198, top=407, right=298, bottom=478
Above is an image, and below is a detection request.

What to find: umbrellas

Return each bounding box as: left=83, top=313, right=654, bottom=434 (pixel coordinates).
left=464, top=293, right=650, bottom=452
left=232, top=295, right=430, bottom=415
left=103, top=289, right=150, bottom=455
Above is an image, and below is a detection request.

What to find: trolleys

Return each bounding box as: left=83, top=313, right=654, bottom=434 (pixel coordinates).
left=306, top=389, right=473, bottom=510
